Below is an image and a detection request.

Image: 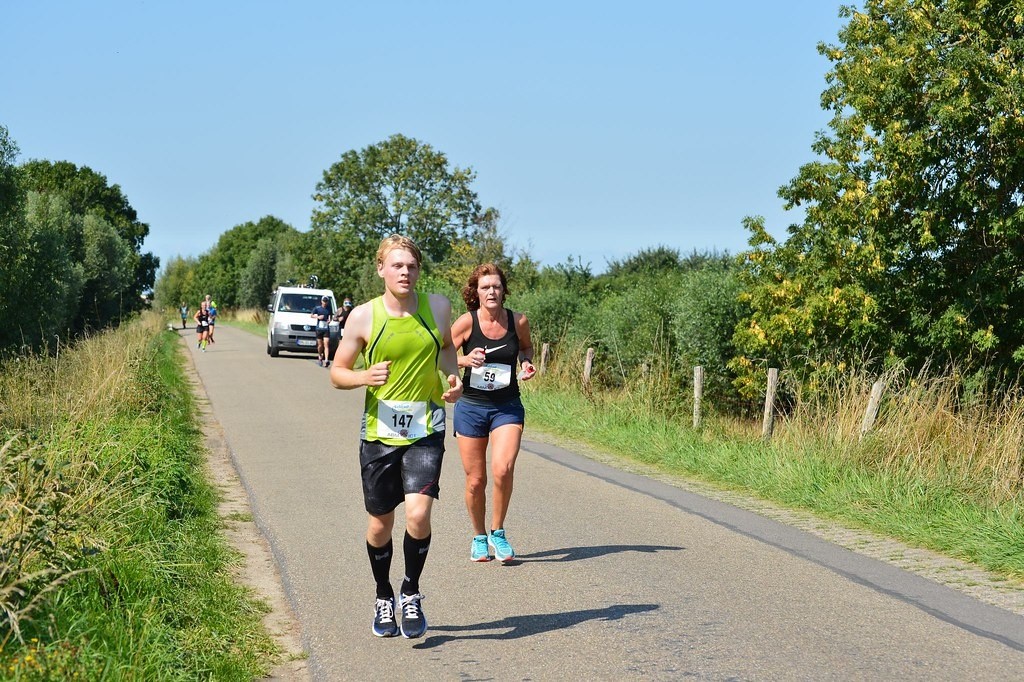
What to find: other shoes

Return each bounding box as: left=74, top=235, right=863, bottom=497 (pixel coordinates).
left=207, top=338, right=215, bottom=345
left=197, top=343, right=206, bottom=352
left=183, top=325, right=186, bottom=329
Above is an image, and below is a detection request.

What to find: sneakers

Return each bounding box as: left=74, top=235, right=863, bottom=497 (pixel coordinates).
left=373, top=595, right=401, bottom=637
left=470, top=529, right=515, bottom=567
left=319, top=354, right=330, bottom=368
left=398, top=591, right=428, bottom=639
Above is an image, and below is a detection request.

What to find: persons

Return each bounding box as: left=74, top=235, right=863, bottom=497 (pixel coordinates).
left=193, top=295, right=218, bottom=353
left=179, top=302, right=189, bottom=329
left=450, top=263, right=537, bottom=564
left=311, top=295, right=353, bottom=367
left=331, top=236, right=463, bottom=638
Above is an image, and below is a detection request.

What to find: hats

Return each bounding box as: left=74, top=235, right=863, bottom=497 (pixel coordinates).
left=344, top=301, right=352, bottom=307
left=321, top=296, right=330, bottom=301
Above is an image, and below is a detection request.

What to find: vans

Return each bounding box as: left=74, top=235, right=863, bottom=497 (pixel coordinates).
left=268, top=286, right=341, bottom=359
